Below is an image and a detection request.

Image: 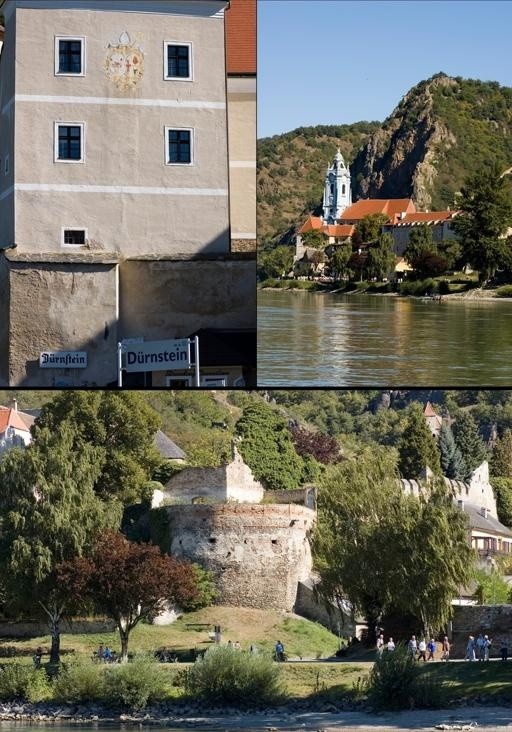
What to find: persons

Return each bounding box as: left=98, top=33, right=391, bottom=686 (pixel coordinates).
left=463, top=633, right=493, bottom=662
left=275, top=641, right=284, bottom=662
left=424, top=291, right=444, bottom=302
left=387, top=637, right=395, bottom=651
left=225, top=640, right=241, bottom=652
left=32, top=646, right=42, bottom=664
left=408, top=635, right=436, bottom=661
left=500, top=642, right=509, bottom=660
left=302, top=274, right=332, bottom=283
left=341, top=640, right=348, bottom=658
left=440, top=636, right=450, bottom=662
left=376, top=633, right=385, bottom=660
left=98, top=646, right=113, bottom=662
